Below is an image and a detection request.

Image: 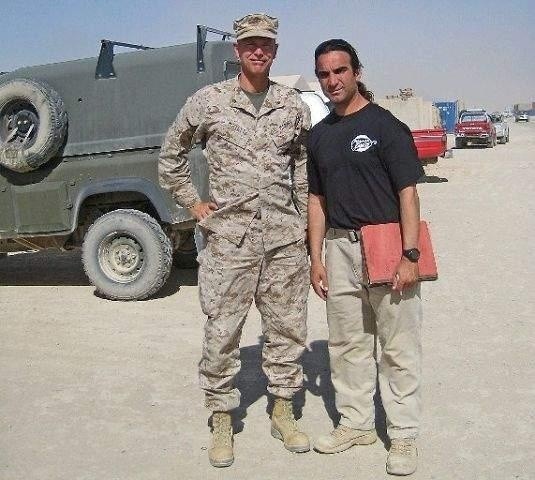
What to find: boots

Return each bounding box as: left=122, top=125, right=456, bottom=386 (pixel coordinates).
left=0, top=23, right=242, bottom=301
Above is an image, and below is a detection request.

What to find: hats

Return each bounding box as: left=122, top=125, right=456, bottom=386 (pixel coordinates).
left=515, top=111, right=529, bottom=122
left=454, top=109, right=509, bottom=147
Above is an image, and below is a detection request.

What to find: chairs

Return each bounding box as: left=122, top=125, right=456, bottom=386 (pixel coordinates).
left=313, top=424, right=377, bottom=454
left=387, top=438, right=418, bottom=475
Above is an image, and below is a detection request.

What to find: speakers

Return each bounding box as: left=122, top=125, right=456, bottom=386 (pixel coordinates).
left=208, top=412, right=233, bottom=467
left=271, top=398, right=310, bottom=452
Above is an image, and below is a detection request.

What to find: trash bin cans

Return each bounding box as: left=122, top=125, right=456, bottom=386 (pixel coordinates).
left=403, top=248, right=420, bottom=262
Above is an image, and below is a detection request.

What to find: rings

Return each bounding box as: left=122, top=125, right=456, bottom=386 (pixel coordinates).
left=396, top=272, right=403, bottom=297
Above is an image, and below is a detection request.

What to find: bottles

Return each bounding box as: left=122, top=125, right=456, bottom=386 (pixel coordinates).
left=233, top=13, right=278, bottom=41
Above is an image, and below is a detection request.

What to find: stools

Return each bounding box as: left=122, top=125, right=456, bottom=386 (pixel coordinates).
left=327, top=228, right=361, bottom=242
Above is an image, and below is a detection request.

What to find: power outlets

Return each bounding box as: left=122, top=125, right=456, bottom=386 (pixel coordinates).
left=303, top=90, right=447, bottom=164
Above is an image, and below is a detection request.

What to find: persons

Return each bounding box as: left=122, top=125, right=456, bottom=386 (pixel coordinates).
left=158, top=12, right=311, bottom=468
left=306, top=39, right=425, bottom=476
